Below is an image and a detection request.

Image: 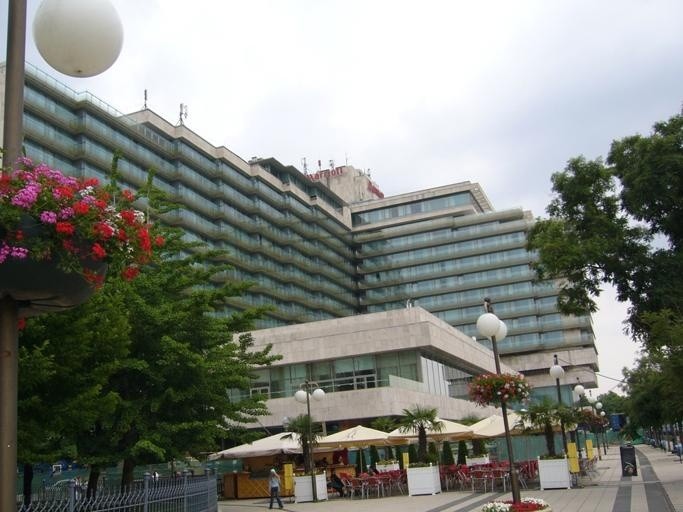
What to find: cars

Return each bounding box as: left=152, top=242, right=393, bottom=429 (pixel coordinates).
left=38, top=477, right=102, bottom=501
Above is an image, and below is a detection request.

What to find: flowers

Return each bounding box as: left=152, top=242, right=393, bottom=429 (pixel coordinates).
left=0, top=146, right=165, bottom=290
left=468, top=373, right=533, bottom=409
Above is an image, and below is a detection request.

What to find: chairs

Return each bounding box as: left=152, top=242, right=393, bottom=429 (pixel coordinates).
left=327, top=461, right=537, bottom=500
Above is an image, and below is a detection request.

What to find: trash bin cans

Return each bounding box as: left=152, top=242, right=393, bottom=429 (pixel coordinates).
left=620, top=443, right=637, bottom=476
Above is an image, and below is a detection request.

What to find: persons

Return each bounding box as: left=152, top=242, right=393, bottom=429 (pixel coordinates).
left=267, top=469, right=283, bottom=510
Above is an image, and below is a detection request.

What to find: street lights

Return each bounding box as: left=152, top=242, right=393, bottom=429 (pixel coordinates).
left=295, top=381, right=326, bottom=501
left=474, top=297, right=520, bottom=504
left=574, top=377, right=610, bottom=475
left=1, top=0, right=124, bottom=512
left=549, top=354, right=569, bottom=458
